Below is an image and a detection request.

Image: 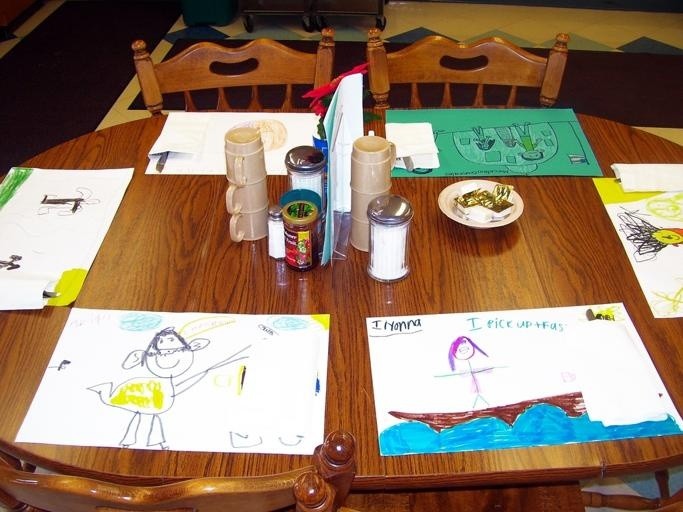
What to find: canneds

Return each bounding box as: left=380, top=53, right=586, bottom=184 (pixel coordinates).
left=282, top=200, right=318, bottom=272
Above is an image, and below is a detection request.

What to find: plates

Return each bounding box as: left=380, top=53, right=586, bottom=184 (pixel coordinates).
left=437, top=179, right=525, bottom=230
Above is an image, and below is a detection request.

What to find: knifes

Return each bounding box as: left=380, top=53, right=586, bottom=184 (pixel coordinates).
left=155, top=152, right=170, bottom=175
left=403, top=157, right=412, bottom=172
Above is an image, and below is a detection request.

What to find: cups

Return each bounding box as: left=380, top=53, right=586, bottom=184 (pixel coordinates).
left=349, top=137, right=396, bottom=253
left=222, top=126, right=268, bottom=243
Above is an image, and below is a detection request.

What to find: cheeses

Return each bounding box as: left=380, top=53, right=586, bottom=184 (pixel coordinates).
left=453, top=184, right=514, bottom=223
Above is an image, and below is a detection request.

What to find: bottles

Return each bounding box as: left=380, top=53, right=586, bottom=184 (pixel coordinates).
left=366, top=194, right=411, bottom=283
left=265, top=147, right=323, bottom=272
